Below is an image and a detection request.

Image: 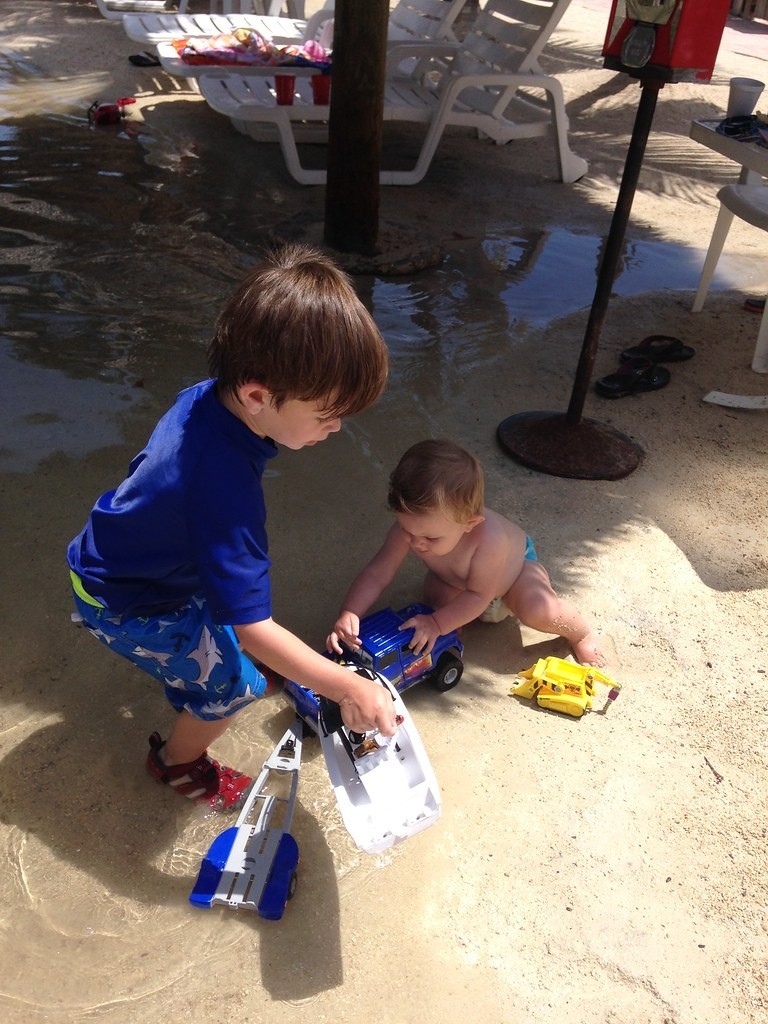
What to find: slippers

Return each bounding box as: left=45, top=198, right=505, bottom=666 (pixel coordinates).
left=129, top=51, right=161, bottom=67
left=595, top=365, right=672, bottom=399
left=620, top=333, right=696, bottom=363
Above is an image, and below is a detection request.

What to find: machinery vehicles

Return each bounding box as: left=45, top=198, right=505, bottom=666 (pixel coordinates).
left=508, top=654, right=624, bottom=719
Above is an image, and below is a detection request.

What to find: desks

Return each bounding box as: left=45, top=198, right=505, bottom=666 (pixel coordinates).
left=690, top=117, right=768, bottom=179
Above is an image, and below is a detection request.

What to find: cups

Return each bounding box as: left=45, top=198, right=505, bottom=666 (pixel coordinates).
left=273, top=74, right=296, bottom=106
left=310, top=74, right=331, bottom=106
left=726, top=77, right=765, bottom=119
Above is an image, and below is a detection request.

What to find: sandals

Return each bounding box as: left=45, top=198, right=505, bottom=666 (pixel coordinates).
left=146, top=731, right=258, bottom=812
left=252, top=662, right=286, bottom=698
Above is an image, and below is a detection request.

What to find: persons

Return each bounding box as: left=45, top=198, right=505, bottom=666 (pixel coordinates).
left=64, top=237, right=398, bottom=815
left=321, top=438, right=605, bottom=671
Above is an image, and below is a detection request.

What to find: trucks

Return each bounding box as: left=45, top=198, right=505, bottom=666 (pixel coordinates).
left=280, top=602, right=466, bottom=741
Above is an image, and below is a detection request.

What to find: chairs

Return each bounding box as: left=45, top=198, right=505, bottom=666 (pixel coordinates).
left=97, top=1, right=588, bottom=186
left=689, top=164, right=768, bottom=374
